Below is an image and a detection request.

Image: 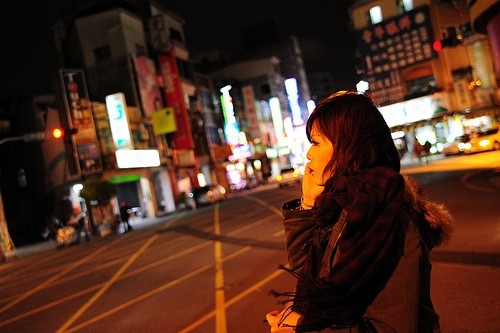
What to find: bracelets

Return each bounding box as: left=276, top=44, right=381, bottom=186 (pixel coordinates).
left=297, top=196, right=313, bottom=210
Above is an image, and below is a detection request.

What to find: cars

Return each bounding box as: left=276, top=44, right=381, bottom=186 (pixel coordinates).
left=278, top=167, right=301, bottom=187
left=441, top=128, right=500, bottom=157
left=194, top=183, right=227, bottom=206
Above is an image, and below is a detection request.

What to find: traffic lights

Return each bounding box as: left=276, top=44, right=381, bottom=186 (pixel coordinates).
left=52, top=128, right=64, bottom=140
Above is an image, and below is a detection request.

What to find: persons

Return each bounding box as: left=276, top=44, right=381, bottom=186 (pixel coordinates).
left=266, top=90, right=455, bottom=333
left=119, top=200, right=133, bottom=234
left=41, top=213, right=91, bottom=247
left=459, top=134, right=469, bottom=142
left=399, top=138, right=432, bottom=167
